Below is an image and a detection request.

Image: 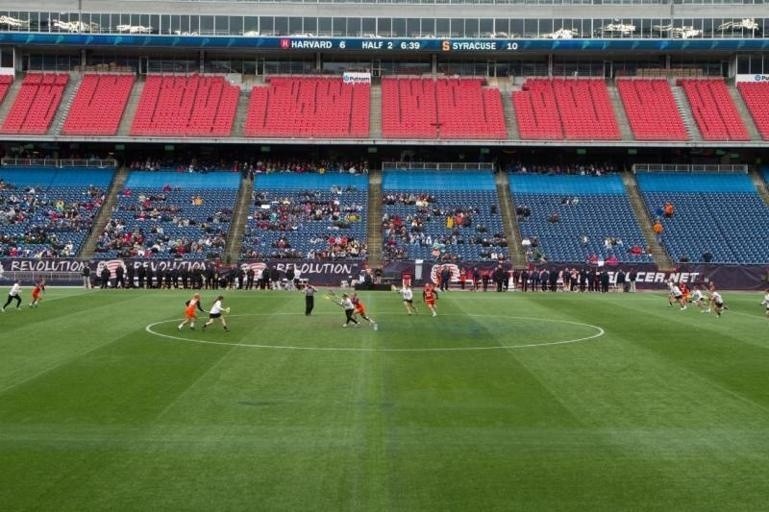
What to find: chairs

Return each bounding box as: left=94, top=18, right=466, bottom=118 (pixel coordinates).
left=0, top=73, right=769, bottom=267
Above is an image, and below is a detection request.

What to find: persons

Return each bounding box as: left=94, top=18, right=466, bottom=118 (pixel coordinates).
left=1, top=141, right=717, bottom=179
left=760, top=287, right=769, bottom=318
left=332, top=294, right=360, bottom=329
left=422, top=283, right=439, bottom=318
left=1, top=171, right=689, bottom=291
left=348, top=292, right=378, bottom=332
left=665, top=278, right=728, bottom=318
left=397, top=282, right=419, bottom=316
left=27, top=278, right=47, bottom=309
left=177, top=293, right=206, bottom=330
left=1, top=279, right=24, bottom=313
left=201, top=296, right=231, bottom=333
left=301, top=282, right=319, bottom=316
